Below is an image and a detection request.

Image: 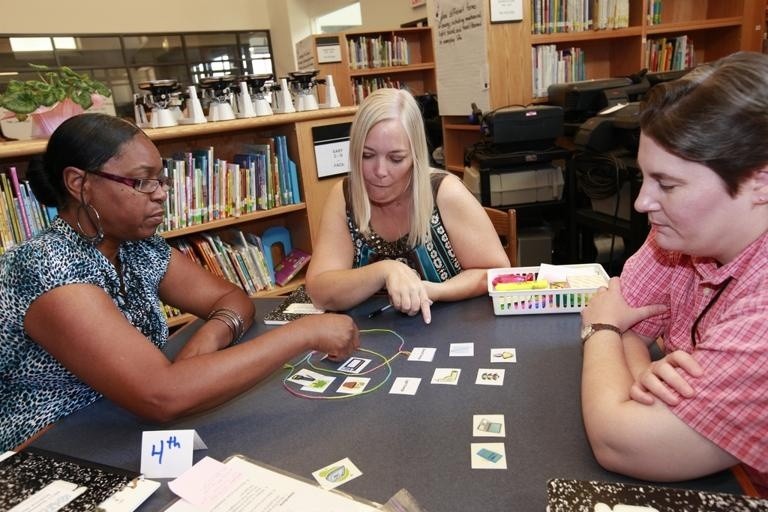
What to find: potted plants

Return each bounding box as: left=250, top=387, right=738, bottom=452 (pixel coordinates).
left=0, top=60, right=119, bottom=145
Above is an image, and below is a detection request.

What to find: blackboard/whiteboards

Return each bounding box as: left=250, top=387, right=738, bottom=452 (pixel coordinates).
left=432, top=0, right=489, bottom=117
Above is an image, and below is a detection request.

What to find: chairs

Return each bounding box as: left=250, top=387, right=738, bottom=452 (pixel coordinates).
left=481, top=204, right=522, bottom=272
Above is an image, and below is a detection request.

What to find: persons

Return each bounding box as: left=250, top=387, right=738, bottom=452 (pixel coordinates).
left=1, top=113, right=360, bottom=454
left=305, top=88, right=512, bottom=325
left=579, top=52, right=768, bottom=500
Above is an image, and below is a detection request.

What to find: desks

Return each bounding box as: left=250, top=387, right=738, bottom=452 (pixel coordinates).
left=0, top=291, right=768, bottom=512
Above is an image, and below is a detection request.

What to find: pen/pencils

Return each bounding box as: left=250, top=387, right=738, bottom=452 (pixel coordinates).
left=369, top=304, right=393, bottom=320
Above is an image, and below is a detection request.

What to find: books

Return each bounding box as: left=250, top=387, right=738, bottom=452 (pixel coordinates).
left=0, top=444, right=161, bottom=512
left=531, top=1, right=694, bottom=99
left=348, top=35, right=410, bottom=105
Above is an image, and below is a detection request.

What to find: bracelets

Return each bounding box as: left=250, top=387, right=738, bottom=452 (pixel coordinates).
left=207, top=309, right=244, bottom=346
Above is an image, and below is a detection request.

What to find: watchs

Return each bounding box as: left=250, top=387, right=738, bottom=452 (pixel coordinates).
left=581, top=323, right=622, bottom=344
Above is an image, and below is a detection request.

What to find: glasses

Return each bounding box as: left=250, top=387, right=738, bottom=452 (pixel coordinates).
left=82, top=163, right=175, bottom=194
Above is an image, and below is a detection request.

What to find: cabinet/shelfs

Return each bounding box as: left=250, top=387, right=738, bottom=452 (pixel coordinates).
left=425, top=1, right=765, bottom=181
left=0, top=100, right=359, bottom=339
left=293, top=19, right=437, bottom=113
